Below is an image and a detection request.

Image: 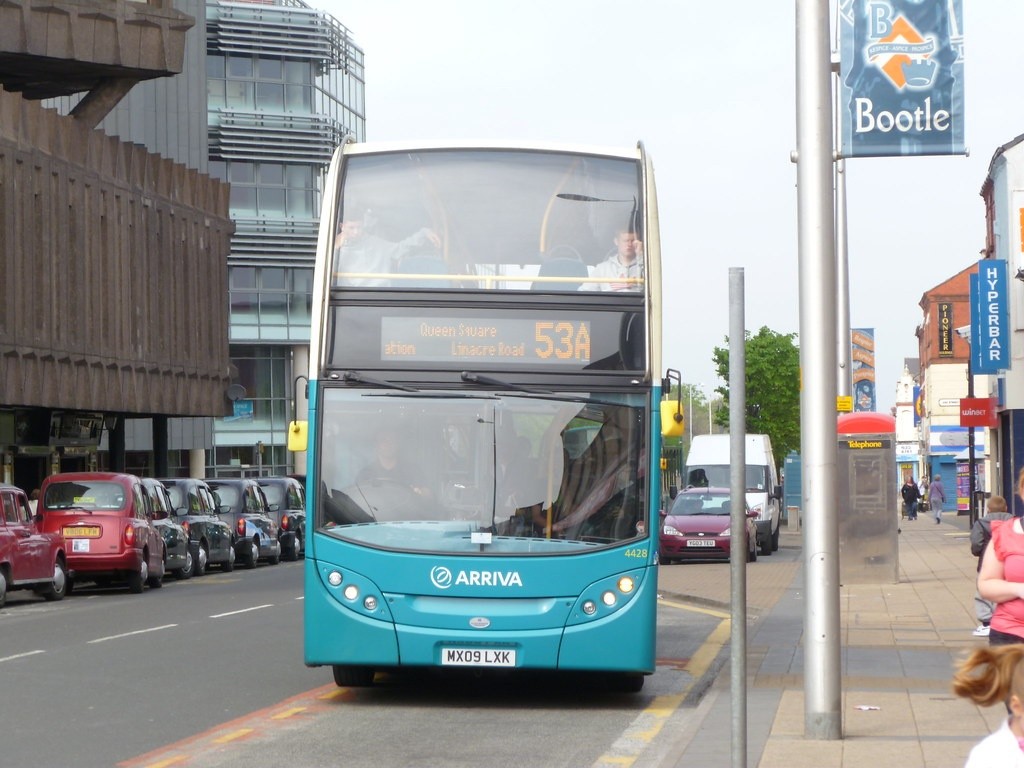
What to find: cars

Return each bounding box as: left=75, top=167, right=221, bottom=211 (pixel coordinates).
left=139, top=476, right=306, bottom=579
left=658, top=484, right=759, bottom=565
left=0, top=483, right=69, bottom=609
left=35, top=471, right=169, bottom=593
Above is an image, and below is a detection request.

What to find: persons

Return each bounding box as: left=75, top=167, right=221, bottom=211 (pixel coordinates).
left=577, top=226, right=644, bottom=292
left=508, top=437, right=635, bottom=539
left=335, top=213, right=441, bottom=287
left=977, top=466, right=1024, bottom=646
left=28, top=489, right=39, bottom=515
left=356, top=429, right=431, bottom=496
left=953, top=643, right=1024, bottom=768
left=902, top=476, right=922, bottom=520
left=969, top=495, right=1013, bottom=636
left=918, top=476, right=926, bottom=513
left=928, top=475, right=946, bottom=523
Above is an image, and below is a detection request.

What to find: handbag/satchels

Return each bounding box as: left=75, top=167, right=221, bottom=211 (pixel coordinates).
left=942, top=497, right=944, bottom=503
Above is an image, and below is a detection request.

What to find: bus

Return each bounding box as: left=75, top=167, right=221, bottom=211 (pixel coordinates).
left=287, top=134, right=685, bottom=691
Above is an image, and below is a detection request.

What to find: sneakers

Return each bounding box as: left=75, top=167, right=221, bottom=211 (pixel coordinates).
left=973, top=625, right=990, bottom=636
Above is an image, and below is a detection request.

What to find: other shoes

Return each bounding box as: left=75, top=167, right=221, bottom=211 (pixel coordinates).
left=936, top=517, right=940, bottom=524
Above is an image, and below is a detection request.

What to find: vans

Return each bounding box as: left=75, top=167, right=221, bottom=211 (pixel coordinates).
left=670, top=434, right=784, bottom=556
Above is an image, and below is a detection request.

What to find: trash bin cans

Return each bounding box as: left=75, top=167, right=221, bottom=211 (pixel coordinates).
left=787, top=506, right=800, bottom=532
